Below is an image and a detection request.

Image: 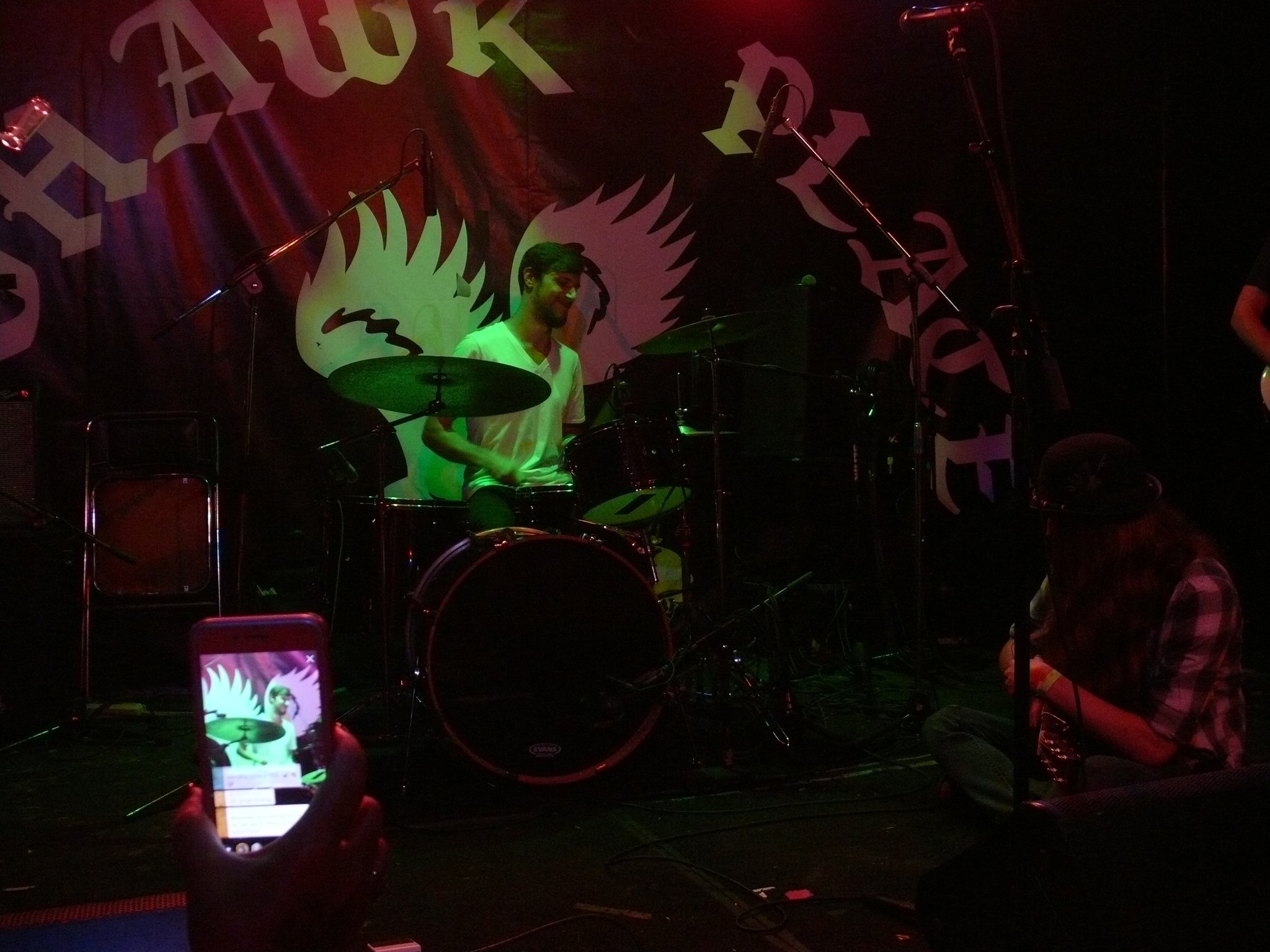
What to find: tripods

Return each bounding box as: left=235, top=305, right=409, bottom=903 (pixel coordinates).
left=690, top=328, right=800, bottom=764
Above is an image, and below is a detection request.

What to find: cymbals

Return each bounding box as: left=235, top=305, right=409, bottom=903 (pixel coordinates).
left=322, top=353, right=550, bottom=418
left=631, top=309, right=780, bottom=355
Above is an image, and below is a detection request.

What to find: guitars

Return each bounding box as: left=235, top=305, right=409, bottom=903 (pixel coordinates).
left=998, top=625, right=1083, bottom=784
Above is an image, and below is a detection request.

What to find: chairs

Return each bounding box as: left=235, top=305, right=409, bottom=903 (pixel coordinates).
left=76, top=408, right=222, bottom=703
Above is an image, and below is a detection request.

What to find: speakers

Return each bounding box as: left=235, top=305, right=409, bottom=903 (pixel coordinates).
left=918, top=763, right=1270, bottom=952
left=0, top=383, right=50, bottom=527
left=0, top=889, right=193, bottom=952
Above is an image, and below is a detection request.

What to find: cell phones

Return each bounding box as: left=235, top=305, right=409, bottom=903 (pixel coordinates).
left=189, top=612, right=332, bottom=855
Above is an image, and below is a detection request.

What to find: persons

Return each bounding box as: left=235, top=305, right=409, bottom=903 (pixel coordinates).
left=1230, top=239, right=1270, bottom=367
left=998, top=434, right=1250, bottom=791
left=172, top=724, right=389, bottom=952
left=422, top=242, right=587, bottom=534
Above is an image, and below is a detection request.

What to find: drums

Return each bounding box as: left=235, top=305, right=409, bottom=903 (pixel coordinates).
left=563, top=417, right=694, bottom=527
left=515, top=484, right=586, bottom=529
left=350, top=497, right=464, bottom=585
left=396, top=525, right=681, bottom=787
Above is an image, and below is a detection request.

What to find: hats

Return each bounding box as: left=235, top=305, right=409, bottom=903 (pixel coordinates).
left=1030, top=432, right=1162, bottom=527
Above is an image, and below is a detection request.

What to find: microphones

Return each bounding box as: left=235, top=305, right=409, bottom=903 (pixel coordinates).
left=600, top=693, right=624, bottom=715
left=900, top=3, right=984, bottom=34
left=421, top=132, right=437, bottom=215
left=752, top=88, right=784, bottom=162
left=612, top=364, right=623, bottom=414
